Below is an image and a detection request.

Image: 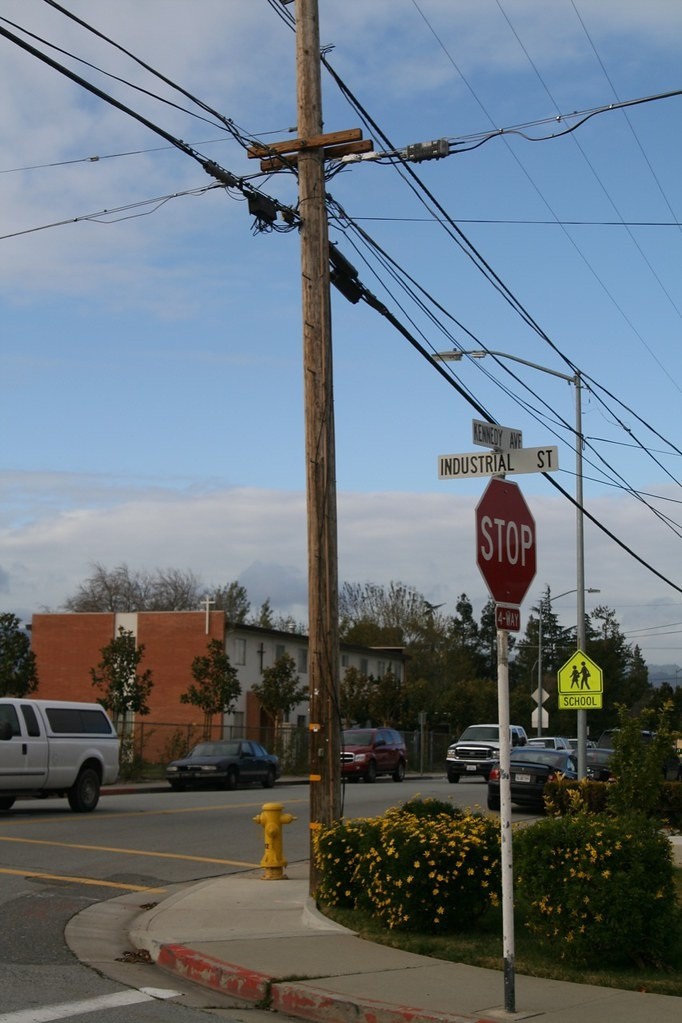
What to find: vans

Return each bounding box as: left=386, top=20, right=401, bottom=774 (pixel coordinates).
left=339, top=727, right=409, bottom=784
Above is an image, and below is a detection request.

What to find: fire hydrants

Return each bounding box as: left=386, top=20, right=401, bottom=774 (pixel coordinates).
left=252, top=802, right=298, bottom=881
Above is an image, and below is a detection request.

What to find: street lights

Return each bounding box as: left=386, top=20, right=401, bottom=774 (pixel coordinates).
left=427, top=344, right=589, bottom=787
left=535, top=587, right=601, bottom=735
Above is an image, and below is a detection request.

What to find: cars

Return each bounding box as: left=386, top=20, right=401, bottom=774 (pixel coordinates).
left=164, top=739, right=283, bottom=791
left=530, top=728, right=674, bottom=780
left=484, top=744, right=576, bottom=810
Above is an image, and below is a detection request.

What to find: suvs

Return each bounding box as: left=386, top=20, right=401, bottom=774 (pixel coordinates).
left=446, top=724, right=530, bottom=785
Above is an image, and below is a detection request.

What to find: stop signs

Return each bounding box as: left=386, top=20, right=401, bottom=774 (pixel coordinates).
left=475, top=474, right=539, bottom=607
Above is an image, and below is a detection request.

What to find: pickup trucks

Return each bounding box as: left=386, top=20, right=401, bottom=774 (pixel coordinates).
left=0, top=696, right=123, bottom=814
